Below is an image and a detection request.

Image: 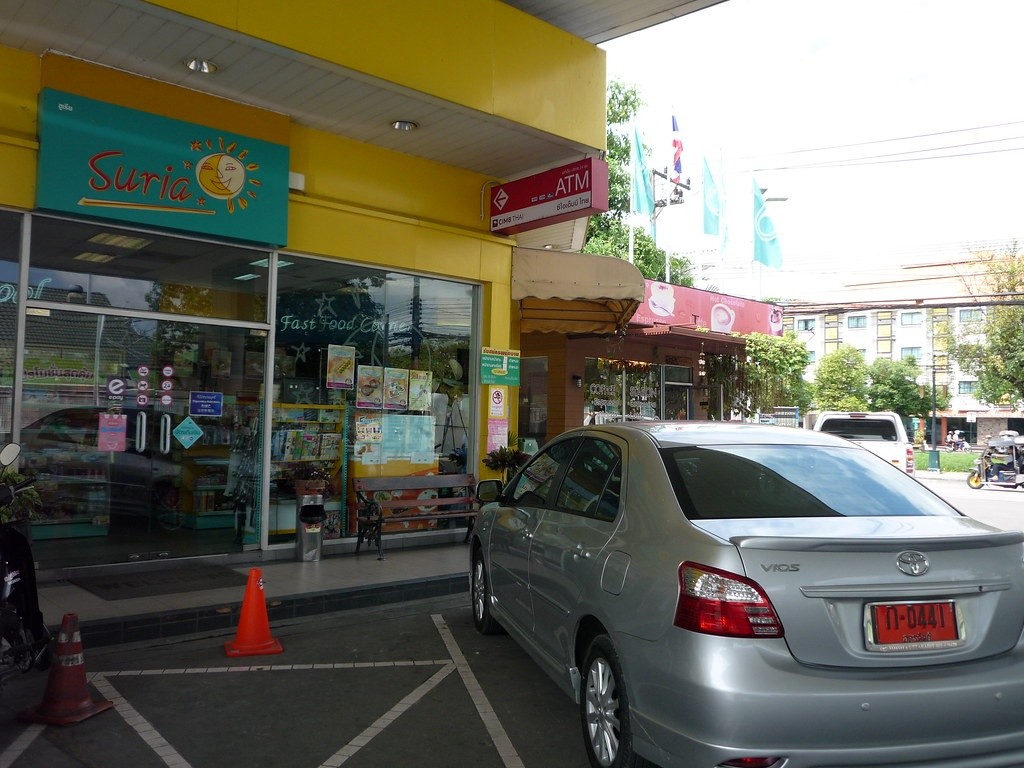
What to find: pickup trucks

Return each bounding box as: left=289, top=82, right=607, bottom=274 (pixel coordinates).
left=812, top=411, right=914, bottom=479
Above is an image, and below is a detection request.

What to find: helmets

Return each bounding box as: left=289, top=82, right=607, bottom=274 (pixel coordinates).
left=954, top=430, right=960, bottom=434
left=948, top=431, right=953, bottom=435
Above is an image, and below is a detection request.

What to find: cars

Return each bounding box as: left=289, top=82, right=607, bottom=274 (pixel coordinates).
left=3, top=407, right=182, bottom=533
left=471, top=424, right=1023, bottom=768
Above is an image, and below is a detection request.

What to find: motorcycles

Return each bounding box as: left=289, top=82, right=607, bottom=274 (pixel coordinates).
left=945, top=439, right=972, bottom=454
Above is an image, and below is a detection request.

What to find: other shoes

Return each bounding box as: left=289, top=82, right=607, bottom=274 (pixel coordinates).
left=989, top=475, right=999, bottom=481
left=32, top=626, right=55, bottom=666
left=15, top=630, right=33, bottom=672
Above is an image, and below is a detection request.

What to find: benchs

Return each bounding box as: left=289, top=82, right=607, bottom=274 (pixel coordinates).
left=352, top=473, right=487, bottom=562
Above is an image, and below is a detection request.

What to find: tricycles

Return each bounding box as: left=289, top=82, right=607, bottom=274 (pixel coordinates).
left=966, top=436, right=1023, bottom=491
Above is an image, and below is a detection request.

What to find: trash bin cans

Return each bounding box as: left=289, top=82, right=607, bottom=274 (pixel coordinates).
left=297, top=494, right=324, bottom=562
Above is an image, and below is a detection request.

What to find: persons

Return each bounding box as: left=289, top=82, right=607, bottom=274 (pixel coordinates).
left=987, top=445, right=1005, bottom=461
left=953, top=429, right=963, bottom=450
left=990, top=445, right=1019, bottom=482
left=946, top=431, right=956, bottom=451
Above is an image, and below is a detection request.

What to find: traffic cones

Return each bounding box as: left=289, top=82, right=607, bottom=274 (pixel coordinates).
left=16, top=613, right=115, bottom=727
left=224, top=568, right=284, bottom=658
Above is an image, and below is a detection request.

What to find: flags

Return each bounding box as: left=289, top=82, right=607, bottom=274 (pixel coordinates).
left=629, top=114, right=656, bottom=215
left=670, top=114, right=683, bottom=184
left=703, top=159, right=721, bottom=237
left=753, top=181, right=783, bottom=269
